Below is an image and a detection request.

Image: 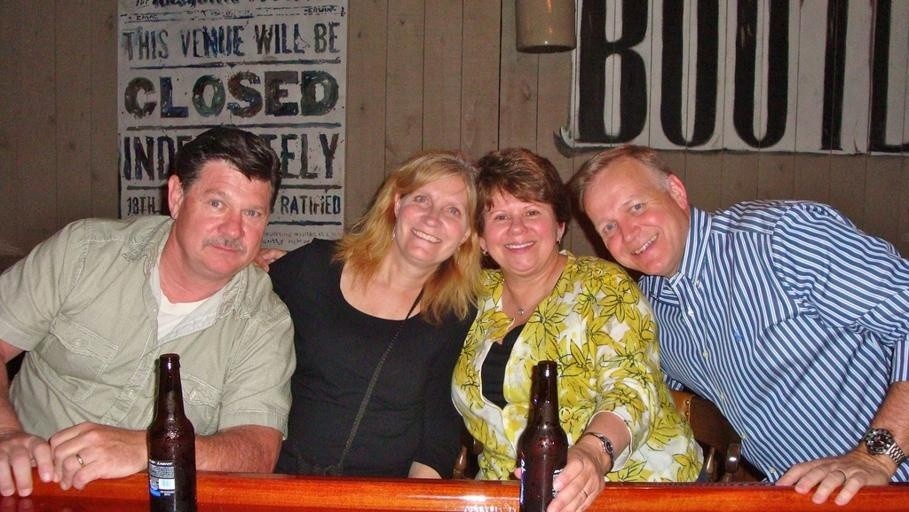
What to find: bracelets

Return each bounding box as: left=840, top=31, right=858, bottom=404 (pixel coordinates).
left=575, top=430, right=615, bottom=477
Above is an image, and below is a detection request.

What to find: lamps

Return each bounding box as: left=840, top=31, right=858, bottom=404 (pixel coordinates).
left=513, top=1, right=576, bottom=53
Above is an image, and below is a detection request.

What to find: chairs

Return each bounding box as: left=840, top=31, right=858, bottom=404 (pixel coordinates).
left=670, top=388, right=739, bottom=481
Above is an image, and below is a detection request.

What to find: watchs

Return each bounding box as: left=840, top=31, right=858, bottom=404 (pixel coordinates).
left=859, top=426, right=905, bottom=467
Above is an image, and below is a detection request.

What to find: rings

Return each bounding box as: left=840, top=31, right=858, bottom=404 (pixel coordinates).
left=74, top=453, right=86, bottom=469
left=580, top=490, right=588, bottom=498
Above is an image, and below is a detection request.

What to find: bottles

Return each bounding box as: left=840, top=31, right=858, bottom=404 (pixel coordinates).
left=518, top=359, right=568, bottom=511
left=146, top=353, right=198, bottom=512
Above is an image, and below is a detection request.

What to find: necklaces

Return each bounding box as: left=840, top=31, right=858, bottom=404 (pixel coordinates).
left=505, top=288, right=546, bottom=315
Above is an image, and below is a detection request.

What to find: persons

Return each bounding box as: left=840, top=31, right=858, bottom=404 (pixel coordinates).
left=0, top=125, right=298, bottom=504
left=249, top=144, right=712, bottom=512
left=565, top=146, right=909, bottom=506
left=266, top=149, right=482, bottom=480
